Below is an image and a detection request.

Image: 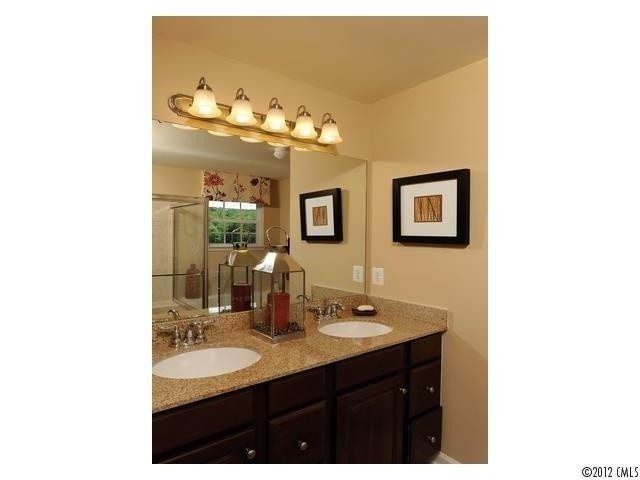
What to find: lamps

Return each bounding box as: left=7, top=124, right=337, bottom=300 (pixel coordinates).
left=227, top=87, right=257, bottom=126
left=290, top=105, right=318, bottom=138
left=260, top=97, right=289, bottom=133
left=318, top=113, right=343, bottom=144
left=190, top=77, right=222, bottom=119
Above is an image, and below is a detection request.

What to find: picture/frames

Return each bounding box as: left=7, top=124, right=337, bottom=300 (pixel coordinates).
left=298, top=188, right=342, bottom=241
left=391, top=169, right=470, bottom=245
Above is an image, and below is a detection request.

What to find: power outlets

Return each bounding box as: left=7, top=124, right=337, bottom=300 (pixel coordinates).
left=353, top=266, right=365, bottom=282
left=372, top=268, right=383, bottom=285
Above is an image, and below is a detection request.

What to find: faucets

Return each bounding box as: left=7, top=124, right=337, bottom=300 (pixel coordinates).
left=159, top=319, right=208, bottom=347
left=167, top=309, right=181, bottom=320
left=296, top=294, right=312, bottom=301
left=306, top=301, right=345, bottom=321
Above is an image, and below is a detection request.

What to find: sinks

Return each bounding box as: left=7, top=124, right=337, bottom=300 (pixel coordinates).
left=153, top=346, right=264, bottom=379
left=319, top=320, right=394, bottom=338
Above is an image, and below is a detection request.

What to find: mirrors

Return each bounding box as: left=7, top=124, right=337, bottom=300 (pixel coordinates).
left=152, top=120, right=367, bottom=325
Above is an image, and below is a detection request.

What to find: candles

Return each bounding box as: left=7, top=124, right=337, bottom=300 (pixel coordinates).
left=268, top=292, right=289, bottom=330
left=231, top=282, right=251, bottom=312
